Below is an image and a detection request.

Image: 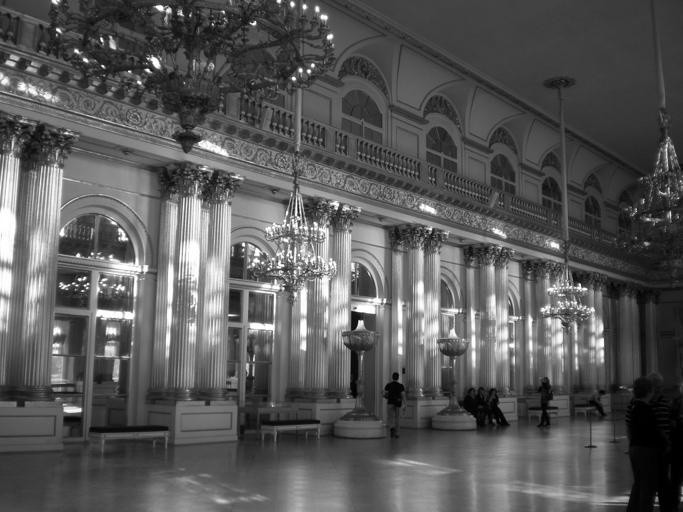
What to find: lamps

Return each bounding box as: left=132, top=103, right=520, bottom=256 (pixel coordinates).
left=619, top=0, right=682, bottom=267
left=539, top=77, right=595, bottom=331
left=245, top=0, right=338, bottom=305
left=46, top=0, right=336, bottom=152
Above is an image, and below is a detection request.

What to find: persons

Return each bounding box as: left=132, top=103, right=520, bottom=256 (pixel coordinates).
left=536, top=377, right=552, bottom=427
left=589, top=390, right=607, bottom=416
left=625, top=371, right=682, bottom=511
left=465, top=385, right=508, bottom=426
left=385, top=373, right=407, bottom=438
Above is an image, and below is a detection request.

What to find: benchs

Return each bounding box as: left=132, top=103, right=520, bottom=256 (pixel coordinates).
left=528, top=406, right=559, bottom=424
left=260, top=419, right=321, bottom=444
left=574, top=404, right=596, bottom=417
left=88, top=424, right=169, bottom=454
